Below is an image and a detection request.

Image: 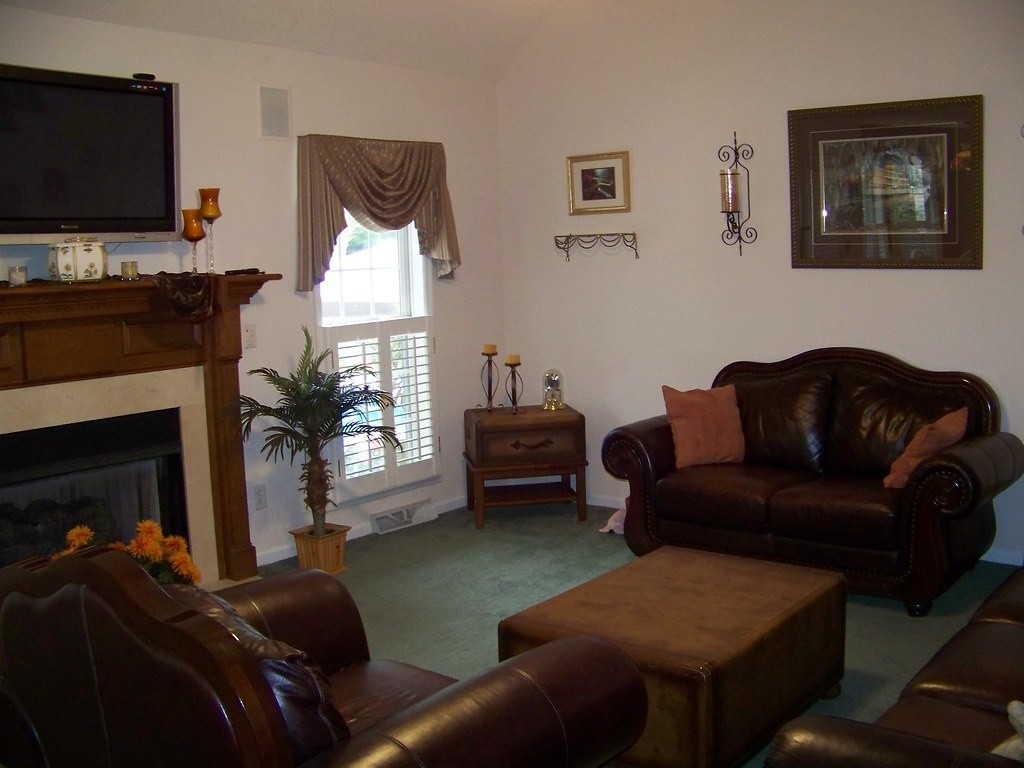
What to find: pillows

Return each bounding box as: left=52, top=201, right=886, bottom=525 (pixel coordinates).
left=662, top=385, right=746, bottom=468
left=883, top=406, right=968, bottom=489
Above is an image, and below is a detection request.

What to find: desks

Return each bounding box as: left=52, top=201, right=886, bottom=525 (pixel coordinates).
left=463, top=404, right=589, bottom=530
left=0, top=269, right=283, bottom=581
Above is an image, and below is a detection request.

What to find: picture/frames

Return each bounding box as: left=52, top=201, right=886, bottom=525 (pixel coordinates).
left=789, top=94, right=984, bottom=270
left=566, top=151, right=631, bottom=216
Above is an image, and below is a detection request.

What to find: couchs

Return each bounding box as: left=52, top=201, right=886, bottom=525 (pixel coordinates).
left=0, top=549, right=650, bottom=768
left=762, top=567, right=1024, bottom=768
left=601, top=345, right=1024, bottom=617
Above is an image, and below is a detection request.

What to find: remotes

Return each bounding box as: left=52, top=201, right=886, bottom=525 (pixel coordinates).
left=225, top=268, right=259, bottom=276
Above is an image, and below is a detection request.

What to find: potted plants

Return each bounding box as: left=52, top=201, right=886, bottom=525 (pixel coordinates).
left=236, top=326, right=402, bottom=574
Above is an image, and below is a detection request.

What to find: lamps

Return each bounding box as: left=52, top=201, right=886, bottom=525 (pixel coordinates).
left=718, top=131, right=758, bottom=256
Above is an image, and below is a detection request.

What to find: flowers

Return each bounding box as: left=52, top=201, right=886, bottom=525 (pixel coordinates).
left=48, top=520, right=201, bottom=587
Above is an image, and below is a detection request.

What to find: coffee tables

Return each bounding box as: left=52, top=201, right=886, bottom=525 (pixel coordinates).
left=497, top=546, right=848, bottom=768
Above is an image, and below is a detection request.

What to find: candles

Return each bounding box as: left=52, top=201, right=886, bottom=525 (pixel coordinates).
left=507, top=355, right=520, bottom=362
left=484, top=344, right=497, bottom=352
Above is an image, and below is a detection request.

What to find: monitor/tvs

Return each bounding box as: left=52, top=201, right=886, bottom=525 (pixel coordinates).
left=0, top=64, right=183, bottom=244
